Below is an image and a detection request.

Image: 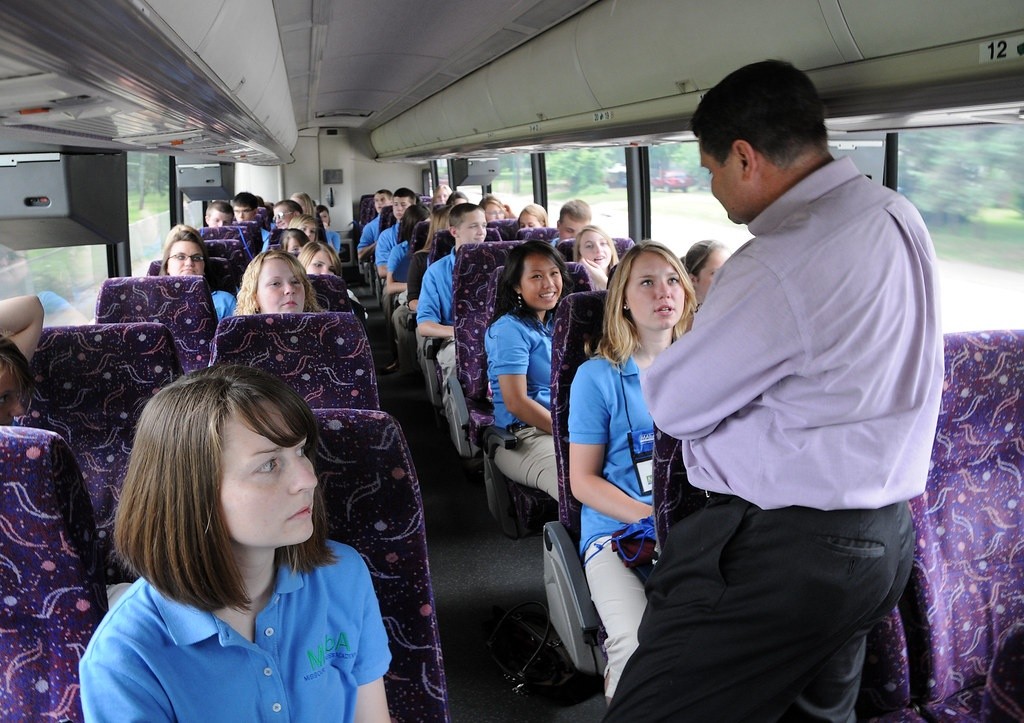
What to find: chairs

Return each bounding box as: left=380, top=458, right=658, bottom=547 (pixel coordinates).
left=198, top=226, right=253, bottom=258
left=484, top=252, right=486, bottom=253
left=307, top=272, right=353, bottom=314
left=94, top=275, right=217, bottom=370
left=357, top=193, right=374, bottom=293
left=13, top=321, right=185, bottom=570
left=207, top=314, right=380, bottom=410
left=487, top=218, right=519, bottom=240
left=911, top=328, right=1024, bottom=723
left=416, top=227, right=503, bottom=409
left=557, top=236, right=636, bottom=261
left=374, top=206, right=392, bottom=308
left=255, top=214, right=263, bottom=226
left=0, top=425, right=110, bottom=723
left=269, top=227, right=283, bottom=246
left=516, top=227, right=558, bottom=241
left=477, top=262, right=596, bottom=542
left=257, top=207, right=268, bottom=221
left=436, top=240, right=564, bottom=490
left=206, top=238, right=247, bottom=282
left=146, top=257, right=237, bottom=297
left=650, top=423, right=927, bottom=723
left=310, top=407, right=450, bottom=723
left=409, top=220, right=430, bottom=253
left=234, top=219, right=264, bottom=248
left=539, top=286, right=610, bottom=681
left=419, top=196, right=434, bottom=208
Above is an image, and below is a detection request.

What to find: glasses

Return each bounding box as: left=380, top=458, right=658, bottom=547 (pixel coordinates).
left=167, top=253, right=204, bottom=263
left=273, top=211, right=294, bottom=222
left=234, top=209, right=253, bottom=216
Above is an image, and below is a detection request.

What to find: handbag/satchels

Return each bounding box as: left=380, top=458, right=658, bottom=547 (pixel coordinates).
left=609, top=517, right=659, bottom=565
left=479, top=599, right=585, bottom=705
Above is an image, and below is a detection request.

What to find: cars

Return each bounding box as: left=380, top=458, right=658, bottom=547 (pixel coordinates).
left=608, top=163, right=693, bottom=192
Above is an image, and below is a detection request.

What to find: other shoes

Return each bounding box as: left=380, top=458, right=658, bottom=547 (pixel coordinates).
left=382, top=357, right=399, bottom=374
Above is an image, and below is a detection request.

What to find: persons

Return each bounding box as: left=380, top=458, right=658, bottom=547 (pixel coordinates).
left=158, top=184, right=737, bottom=506
left=0, top=242, right=107, bottom=329
left=0, top=295, right=44, bottom=426
left=599, top=60, right=944, bottom=723
left=78, top=363, right=394, bottom=723
left=569, top=241, right=698, bottom=708
